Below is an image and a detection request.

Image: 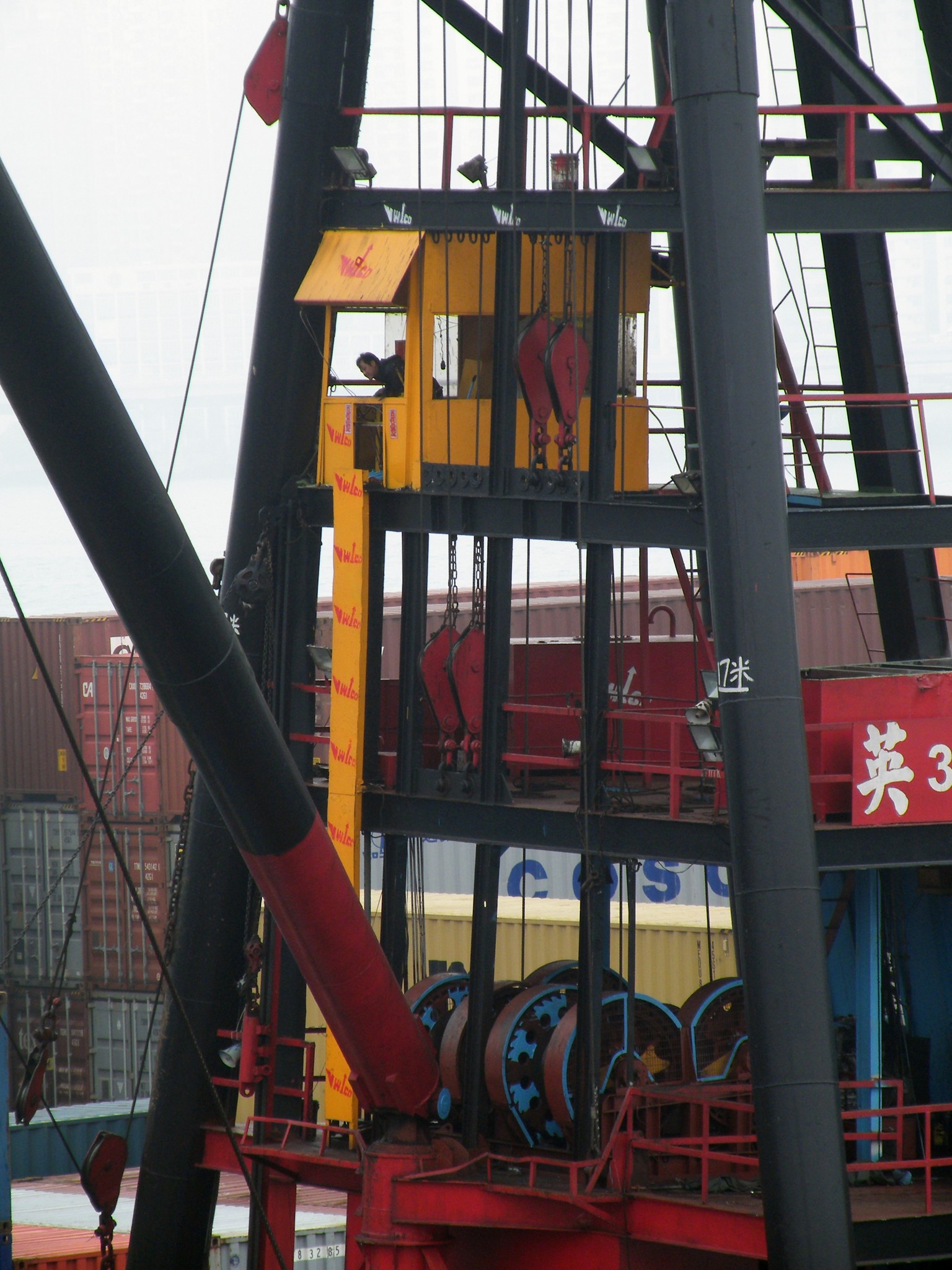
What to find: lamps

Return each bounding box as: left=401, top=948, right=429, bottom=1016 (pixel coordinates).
left=457, top=154, right=489, bottom=190
left=687, top=723, right=723, bottom=753
left=671, top=469, right=702, bottom=498
left=306, top=644, right=333, bottom=673
left=330, top=146, right=377, bottom=188
left=626, top=145, right=676, bottom=191
left=699, top=669, right=721, bottom=701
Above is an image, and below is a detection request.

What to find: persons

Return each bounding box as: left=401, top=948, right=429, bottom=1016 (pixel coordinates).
left=356, top=352, right=443, bottom=399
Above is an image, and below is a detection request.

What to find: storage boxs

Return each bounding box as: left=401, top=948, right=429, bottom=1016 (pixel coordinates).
left=0, top=543, right=952, bottom=1270
